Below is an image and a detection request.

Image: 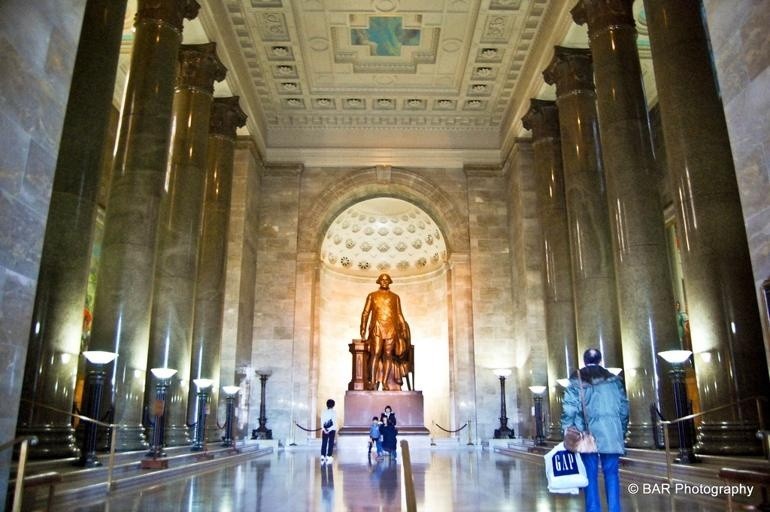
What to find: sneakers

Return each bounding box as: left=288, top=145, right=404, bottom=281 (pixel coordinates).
left=320, top=455, right=333, bottom=460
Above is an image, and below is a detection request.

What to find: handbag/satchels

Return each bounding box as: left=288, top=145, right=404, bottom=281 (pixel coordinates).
left=561, top=423, right=599, bottom=454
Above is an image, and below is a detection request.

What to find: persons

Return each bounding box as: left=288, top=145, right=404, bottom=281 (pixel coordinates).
left=674, top=301, right=690, bottom=350
left=360, top=274, right=407, bottom=390
left=375, top=419, right=399, bottom=460
left=319, top=398, right=337, bottom=465
left=380, top=405, right=397, bottom=427
left=320, top=463, right=336, bottom=511
left=367, top=417, right=383, bottom=454
left=560, top=348, right=632, bottom=512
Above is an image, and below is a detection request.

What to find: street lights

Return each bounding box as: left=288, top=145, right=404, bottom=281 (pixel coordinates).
left=222, top=385, right=241, bottom=449
left=658, top=349, right=698, bottom=465
left=192, top=378, right=213, bottom=453
left=528, top=385, right=547, bottom=445
left=252, top=366, right=273, bottom=440
left=145, top=367, right=178, bottom=458
left=493, top=368, right=514, bottom=440
left=69, top=349, right=120, bottom=469
